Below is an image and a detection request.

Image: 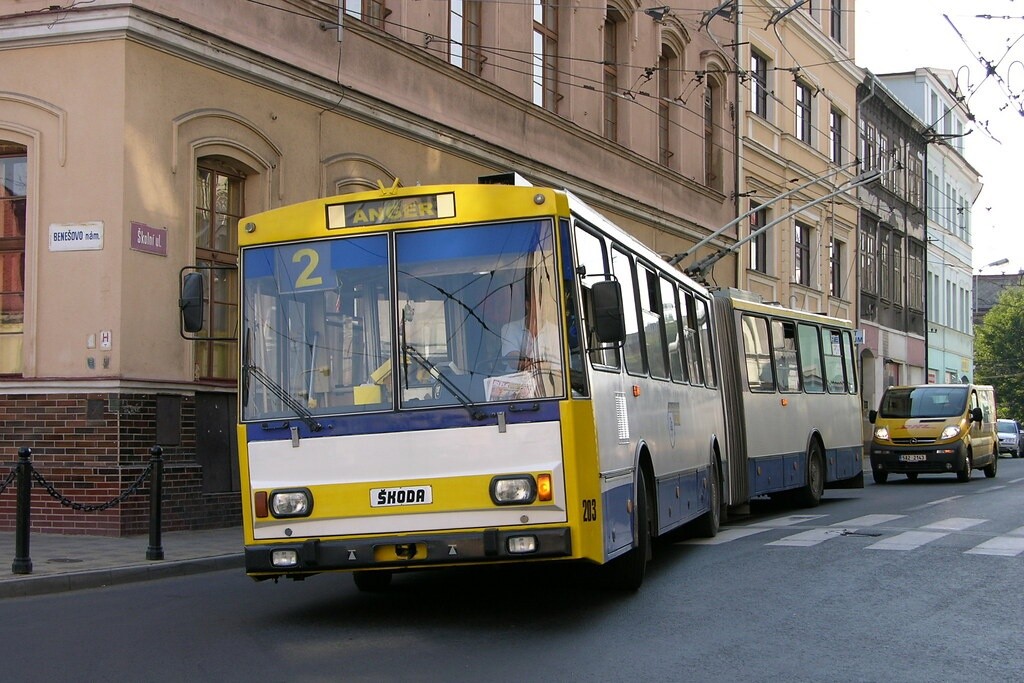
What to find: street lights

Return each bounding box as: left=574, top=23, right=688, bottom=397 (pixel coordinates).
left=975, top=258, right=1010, bottom=312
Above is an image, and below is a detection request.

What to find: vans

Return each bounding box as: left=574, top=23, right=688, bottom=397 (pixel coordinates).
left=867, top=384, right=999, bottom=482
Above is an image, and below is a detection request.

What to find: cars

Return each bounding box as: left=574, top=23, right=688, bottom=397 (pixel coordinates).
left=996, top=418, right=1024, bottom=457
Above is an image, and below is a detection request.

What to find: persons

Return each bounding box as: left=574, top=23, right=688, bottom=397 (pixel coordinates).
left=501, top=286, right=573, bottom=372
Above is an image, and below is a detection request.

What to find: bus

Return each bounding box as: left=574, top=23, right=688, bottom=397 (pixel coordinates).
left=176, top=173, right=865, bottom=589
